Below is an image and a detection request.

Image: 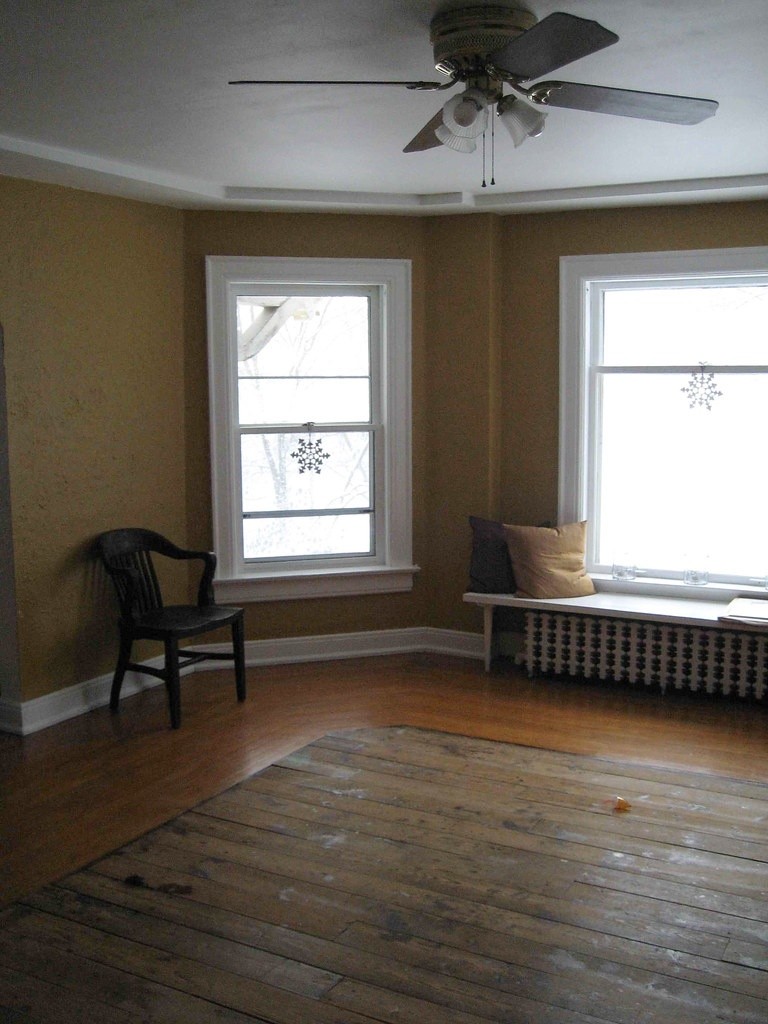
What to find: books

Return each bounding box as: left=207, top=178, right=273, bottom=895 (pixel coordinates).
left=718, top=597, right=768, bottom=626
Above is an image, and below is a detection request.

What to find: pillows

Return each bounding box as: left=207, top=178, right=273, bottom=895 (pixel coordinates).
left=466, top=515, right=550, bottom=593
left=501, top=520, right=598, bottom=598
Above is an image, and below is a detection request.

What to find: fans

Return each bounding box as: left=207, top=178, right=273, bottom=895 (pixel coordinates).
left=228, top=7, right=719, bottom=153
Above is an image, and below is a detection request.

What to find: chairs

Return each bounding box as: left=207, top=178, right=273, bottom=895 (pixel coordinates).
left=91, top=528, right=246, bottom=729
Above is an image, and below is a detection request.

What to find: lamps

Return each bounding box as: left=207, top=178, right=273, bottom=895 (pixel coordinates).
left=435, top=72, right=548, bottom=154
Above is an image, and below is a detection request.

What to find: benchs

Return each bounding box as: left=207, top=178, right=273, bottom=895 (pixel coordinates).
left=462, top=588, right=768, bottom=675
